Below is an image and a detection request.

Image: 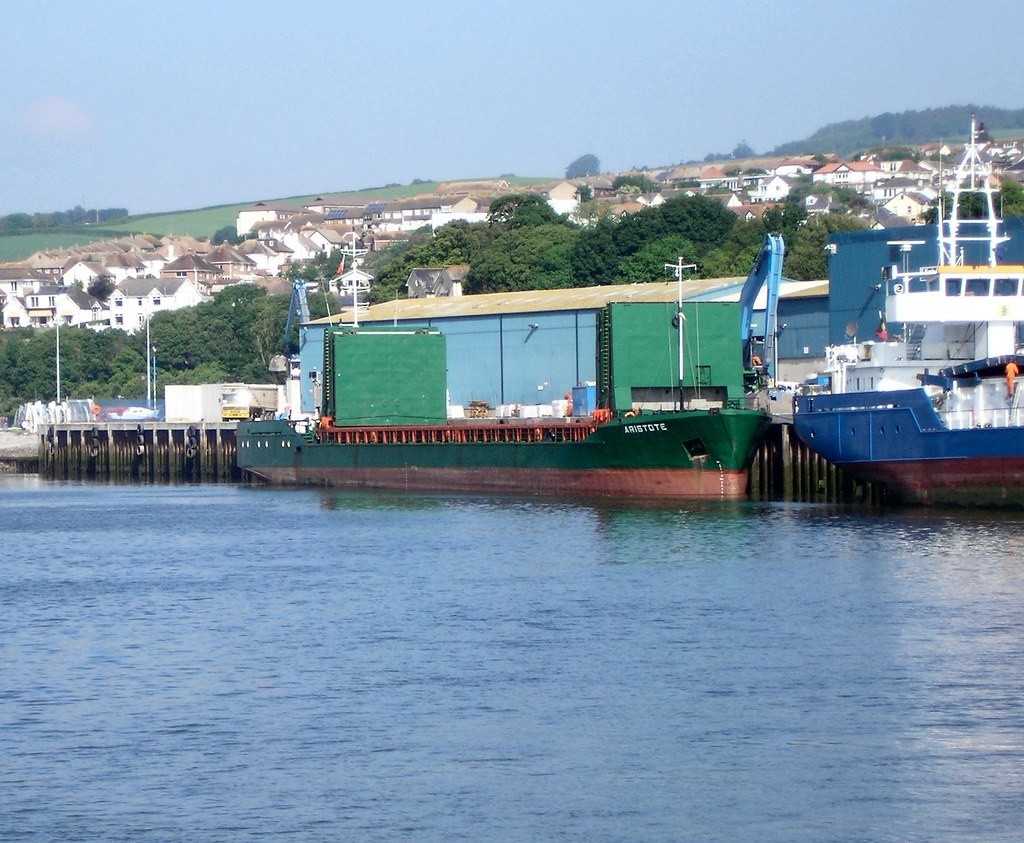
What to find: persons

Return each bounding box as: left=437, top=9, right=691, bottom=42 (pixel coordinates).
left=876, top=312, right=888, bottom=341
left=753, top=354, right=762, bottom=367
left=1006, top=358, right=1018, bottom=397
left=536, top=428, right=541, bottom=443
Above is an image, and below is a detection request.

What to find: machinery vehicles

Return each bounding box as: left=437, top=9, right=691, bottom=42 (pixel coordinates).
left=736, top=231, right=792, bottom=401
left=268, top=278, right=311, bottom=385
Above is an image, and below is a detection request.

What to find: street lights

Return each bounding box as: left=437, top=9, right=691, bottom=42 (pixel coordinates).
left=51, top=314, right=65, bottom=407
left=142, top=312, right=156, bottom=407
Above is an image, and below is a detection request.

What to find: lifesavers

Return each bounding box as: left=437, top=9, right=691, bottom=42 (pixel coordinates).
left=90, top=427, right=99, bottom=457
left=135, top=424, right=145, bottom=455
left=92, top=405, right=100, bottom=415
left=186, top=426, right=197, bottom=458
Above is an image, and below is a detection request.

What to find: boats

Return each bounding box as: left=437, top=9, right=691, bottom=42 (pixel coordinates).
left=235, top=230, right=773, bottom=501
left=790, top=109, right=1024, bottom=510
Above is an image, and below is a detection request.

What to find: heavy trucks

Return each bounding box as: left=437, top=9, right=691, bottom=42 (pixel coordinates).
left=218, top=383, right=278, bottom=421
